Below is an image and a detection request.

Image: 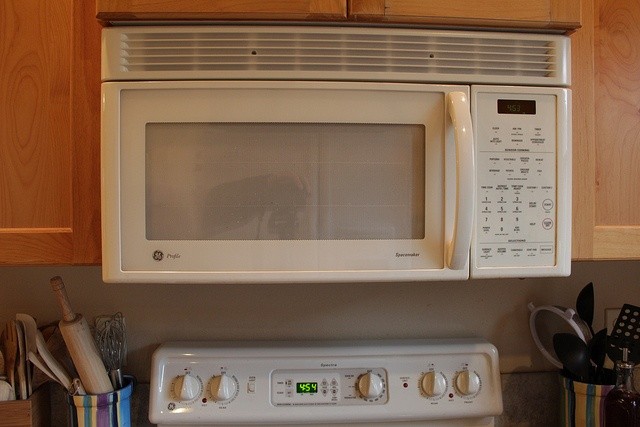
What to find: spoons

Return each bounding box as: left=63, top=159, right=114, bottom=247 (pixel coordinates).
left=551, top=332, right=588, bottom=379
left=575, top=281, right=596, bottom=335
left=589, top=327, right=609, bottom=379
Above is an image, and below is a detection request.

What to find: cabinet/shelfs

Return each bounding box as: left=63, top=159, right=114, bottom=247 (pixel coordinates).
left=0, top=0, right=103, bottom=266
left=349, top=0, right=582, bottom=33
left=571, top=0, right=640, bottom=261
left=95, top=1, right=348, bottom=23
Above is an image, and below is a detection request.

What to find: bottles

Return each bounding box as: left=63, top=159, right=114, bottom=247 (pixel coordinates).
left=604, top=352, right=640, bottom=427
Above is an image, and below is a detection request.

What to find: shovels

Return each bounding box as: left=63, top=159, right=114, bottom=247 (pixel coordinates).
left=607, top=304, right=640, bottom=383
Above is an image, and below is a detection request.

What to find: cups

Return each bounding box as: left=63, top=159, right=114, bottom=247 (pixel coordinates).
left=557, top=372, right=616, bottom=427
left=64, top=375, right=135, bottom=427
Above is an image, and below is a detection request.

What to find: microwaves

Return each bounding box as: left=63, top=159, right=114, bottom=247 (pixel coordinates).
left=100, top=26, right=573, bottom=284
